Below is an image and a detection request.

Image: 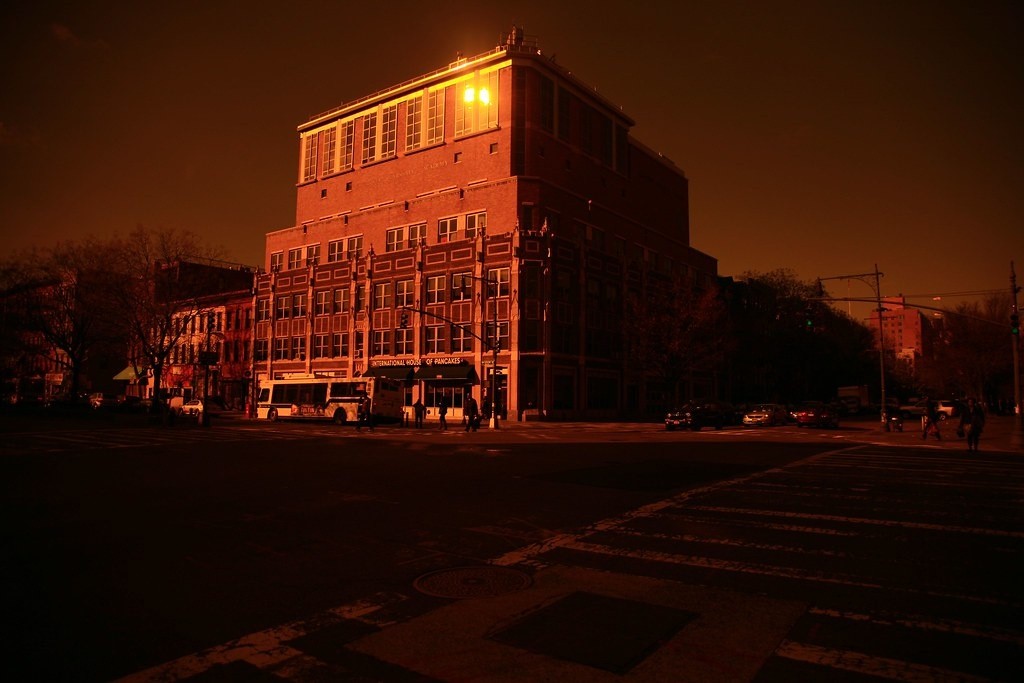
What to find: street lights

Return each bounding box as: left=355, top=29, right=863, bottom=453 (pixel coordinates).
left=815, top=262, right=890, bottom=433
left=458, top=273, right=499, bottom=430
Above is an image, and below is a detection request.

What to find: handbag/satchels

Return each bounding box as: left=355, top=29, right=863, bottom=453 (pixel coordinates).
left=474, top=419, right=481, bottom=429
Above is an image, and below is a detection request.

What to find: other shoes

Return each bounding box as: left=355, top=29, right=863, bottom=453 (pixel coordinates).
left=472, top=429, right=477, bottom=432
left=464, top=429, right=469, bottom=432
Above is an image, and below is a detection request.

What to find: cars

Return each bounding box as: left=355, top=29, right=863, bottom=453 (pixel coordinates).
left=876, top=398, right=900, bottom=414
left=178, top=399, right=222, bottom=419
left=664, top=401, right=857, bottom=432
left=88, top=392, right=141, bottom=414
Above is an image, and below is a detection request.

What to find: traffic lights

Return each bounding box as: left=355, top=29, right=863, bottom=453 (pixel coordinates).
left=1010, top=314, right=1019, bottom=335
left=805, top=307, right=814, bottom=326
left=399, top=312, right=408, bottom=330
left=207, top=311, right=215, bottom=330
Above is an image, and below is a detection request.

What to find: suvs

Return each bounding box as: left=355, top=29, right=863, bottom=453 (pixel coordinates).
left=897, top=396, right=957, bottom=421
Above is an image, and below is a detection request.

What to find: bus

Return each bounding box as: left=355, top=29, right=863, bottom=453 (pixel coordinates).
left=256, top=376, right=405, bottom=425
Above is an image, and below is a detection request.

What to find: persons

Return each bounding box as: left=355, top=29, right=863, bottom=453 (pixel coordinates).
left=462, top=393, right=479, bottom=432
left=353, top=390, right=375, bottom=432
left=958, top=396, right=986, bottom=456
left=438, top=396, right=448, bottom=430
left=412, top=399, right=426, bottom=428
left=915, top=395, right=941, bottom=439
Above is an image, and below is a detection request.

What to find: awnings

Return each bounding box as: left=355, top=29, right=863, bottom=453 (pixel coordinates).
left=362, top=365, right=474, bottom=381
